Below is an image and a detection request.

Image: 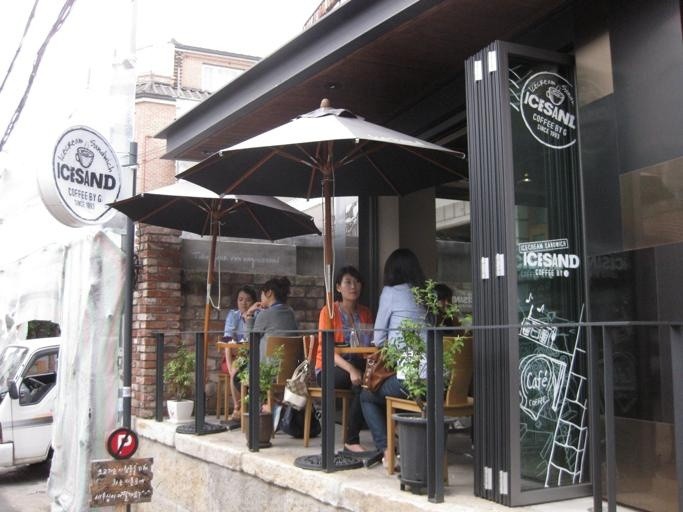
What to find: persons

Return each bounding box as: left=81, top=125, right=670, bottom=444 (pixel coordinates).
left=217, top=287, right=258, bottom=423
left=313, top=265, right=375, bottom=453
left=233, top=274, right=300, bottom=396
left=433, top=282, right=457, bottom=325
left=359, top=247, right=450, bottom=467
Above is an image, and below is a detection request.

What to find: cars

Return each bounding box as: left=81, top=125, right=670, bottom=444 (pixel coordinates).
left=0, top=336, right=64, bottom=477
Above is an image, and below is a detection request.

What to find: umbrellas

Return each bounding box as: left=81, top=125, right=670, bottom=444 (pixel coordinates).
left=103, top=183, right=324, bottom=388
left=171, top=98, right=468, bottom=332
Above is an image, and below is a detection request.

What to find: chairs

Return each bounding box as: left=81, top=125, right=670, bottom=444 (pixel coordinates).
left=216, top=336, right=476, bottom=475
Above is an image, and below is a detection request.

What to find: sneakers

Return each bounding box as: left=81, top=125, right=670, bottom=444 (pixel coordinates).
left=381, top=452, right=400, bottom=472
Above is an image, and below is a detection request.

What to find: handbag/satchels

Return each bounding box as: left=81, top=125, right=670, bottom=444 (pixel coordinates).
left=282, top=360, right=308, bottom=411
left=361, top=359, right=395, bottom=393
left=280, top=406, right=321, bottom=437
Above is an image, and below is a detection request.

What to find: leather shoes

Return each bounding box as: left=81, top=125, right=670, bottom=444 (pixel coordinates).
left=343, top=442, right=354, bottom=452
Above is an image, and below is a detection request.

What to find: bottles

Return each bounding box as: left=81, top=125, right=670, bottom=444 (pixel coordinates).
left=349, top=328, right=359, bottom=348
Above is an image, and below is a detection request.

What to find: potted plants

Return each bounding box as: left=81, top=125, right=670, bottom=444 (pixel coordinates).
left=376, top=281, right=471, bottom=495
left=163, top=341, right=196, bottom=421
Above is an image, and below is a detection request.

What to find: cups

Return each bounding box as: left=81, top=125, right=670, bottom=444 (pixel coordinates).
left=74, top=147, right=94, bottom=168
left=356, top=328, right=371, bottom=347
left=545, top=87, right=565, bottom=106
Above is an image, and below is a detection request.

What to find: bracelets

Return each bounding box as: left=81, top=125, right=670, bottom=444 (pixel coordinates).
left=244, top=312, right=253, bottom=317
